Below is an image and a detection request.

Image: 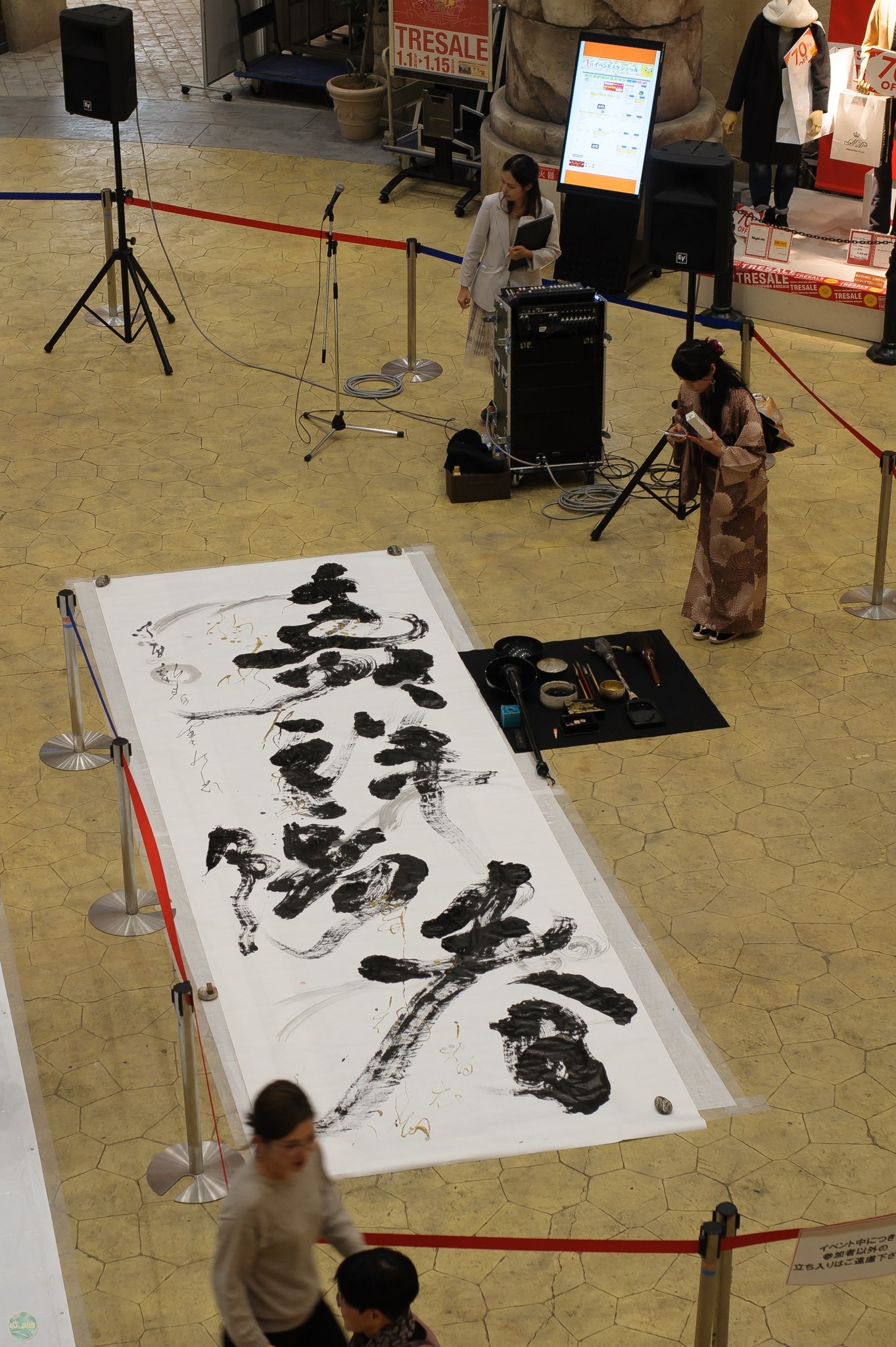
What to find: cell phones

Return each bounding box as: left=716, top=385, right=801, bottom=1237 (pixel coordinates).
left=657, top=428, right=685, bottom=438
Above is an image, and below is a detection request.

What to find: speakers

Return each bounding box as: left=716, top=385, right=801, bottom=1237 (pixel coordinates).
left=645, top=140, right=735, bottom=276
left=59, top=4, right=138, bottom=123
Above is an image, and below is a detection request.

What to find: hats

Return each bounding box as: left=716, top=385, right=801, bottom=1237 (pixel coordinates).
left=443, top=428, right=482, bottom=473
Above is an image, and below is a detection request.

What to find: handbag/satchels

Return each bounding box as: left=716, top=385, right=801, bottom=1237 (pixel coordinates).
left=775, top=22, right=858, bottom=145
left=829, top=87, right=893, bottom=168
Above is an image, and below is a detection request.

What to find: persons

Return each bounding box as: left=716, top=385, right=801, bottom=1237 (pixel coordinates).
left=334, top=1247, right=441, bottom=1347
left=721, top=0, right=831, bottom=227
left=663, top=336, right=794, bottom=644
left=853, top=0, right=896, bottom=238
left=212, top=1080, right=364, bottom=1347
left=458, top=154, right=561, bottom=423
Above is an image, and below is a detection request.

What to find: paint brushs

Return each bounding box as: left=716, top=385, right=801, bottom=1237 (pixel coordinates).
left=586, top=663, right=602, bottom=695
left=582, top=665, right=596, bottom=697
left=572, top=664, right=589, bottom=699
left=502, top=665, right=550, bottom=778
left=575, top=660, right=594, bottom=699
left=595, top=637, right=640, bottom=701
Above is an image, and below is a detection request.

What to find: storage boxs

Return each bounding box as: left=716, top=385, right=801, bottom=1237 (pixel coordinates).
left=445, top=461, right=511, bottom=504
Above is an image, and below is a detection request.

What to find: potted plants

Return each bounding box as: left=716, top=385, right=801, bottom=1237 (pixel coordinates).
left=326, top=0, right=389, bottom=141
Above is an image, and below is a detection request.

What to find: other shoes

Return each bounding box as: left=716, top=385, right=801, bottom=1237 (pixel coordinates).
left=480, top=399, right=496, bottom=422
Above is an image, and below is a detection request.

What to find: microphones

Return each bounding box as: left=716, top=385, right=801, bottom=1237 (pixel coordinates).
left=323, top=182, right=345, bottom=221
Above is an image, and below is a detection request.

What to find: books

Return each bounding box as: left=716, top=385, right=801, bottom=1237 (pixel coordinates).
left=508, top=214, right=554, bottom=272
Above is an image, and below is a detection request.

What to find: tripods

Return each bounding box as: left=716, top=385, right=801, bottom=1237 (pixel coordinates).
left=303, top=214, right=405, bottom=463
left=43, top=122, right=176, bottom=375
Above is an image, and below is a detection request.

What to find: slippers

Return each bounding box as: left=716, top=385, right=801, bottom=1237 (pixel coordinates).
left=692, top=623, right=712, bottom=639
left=710, top=630, right=743, bottom=643
